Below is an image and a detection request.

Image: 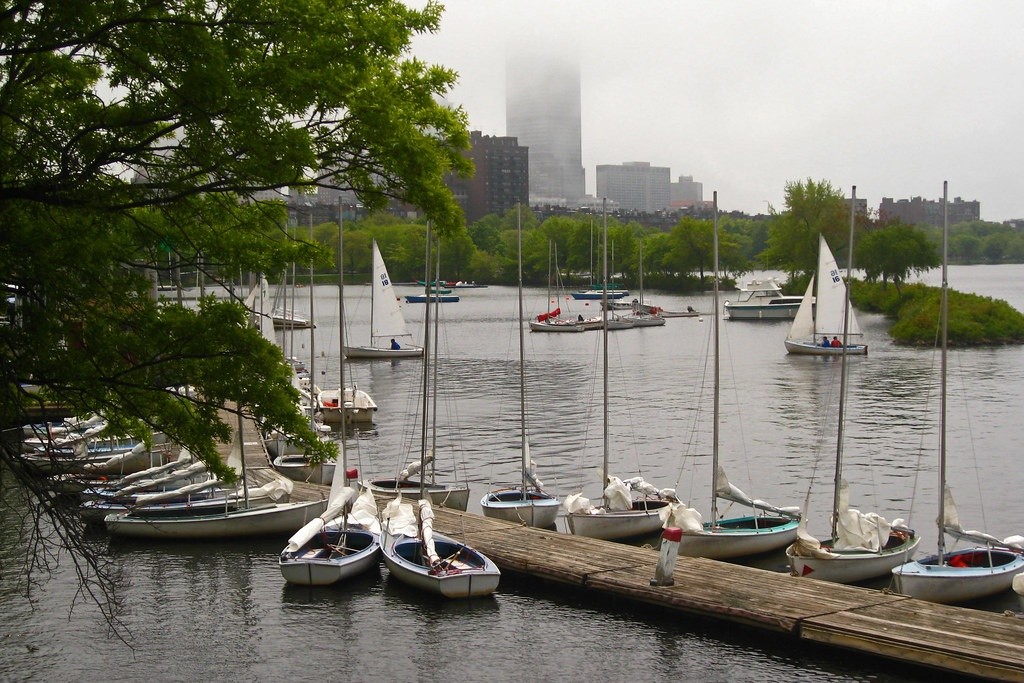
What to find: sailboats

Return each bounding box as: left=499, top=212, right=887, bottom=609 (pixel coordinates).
left=784, top=233, right=869, bottom=355
left=279, top=194, right=382, bottom=585
left=892, top=181, right=1024, bottom=601
left=380, top=218, right=501, bottom=600
left=528, top=219, right=698, bottom=333
left=678, top=191, right=799, bottom=558
left=20, top=196, right=377, bottom=539
left=343, top=238, right=423, bottom=359
left=479, top=202, right=560, bottom=530
left=357, top=220, right=469, bottom=512
left=786, top=185, right=922, bottom=584
left=566, top=197, right=672, bottom=541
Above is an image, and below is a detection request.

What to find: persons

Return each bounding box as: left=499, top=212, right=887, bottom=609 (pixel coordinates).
left=818, top=336, right=830, bottom=347
left=578, top=315, right=584, bottom=321
left=831, top=336, right=842, bottom=347
left=687, top=306, right=693, bottom=312
left=391, top=339, right=400, bottom=350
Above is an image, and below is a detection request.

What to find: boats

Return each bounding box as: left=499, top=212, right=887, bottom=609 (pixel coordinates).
left=444, top=286, right=488, bottom=288
left=405, top=296, right=459, bottom=303
left=425, top=290, right=451, bottom=294
left=725, top=278, right=817, bottom=319
left=416, top=279, right=446, bottom=286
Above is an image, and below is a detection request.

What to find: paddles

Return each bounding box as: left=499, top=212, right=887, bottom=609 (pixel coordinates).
left=326, top=543, right=359, bottom=556
left=445, top=546, right=464, bottom=570
